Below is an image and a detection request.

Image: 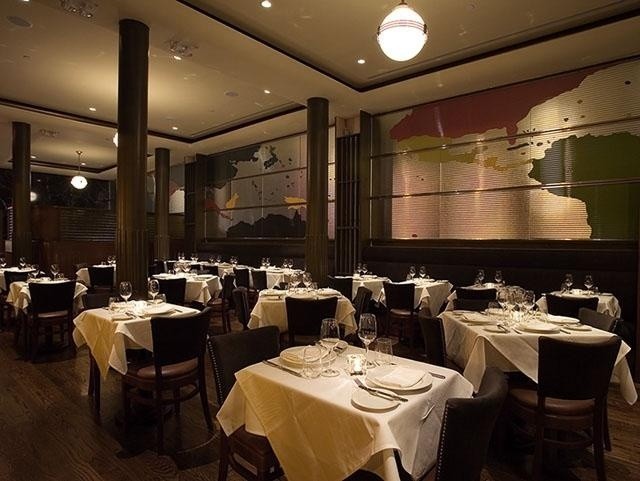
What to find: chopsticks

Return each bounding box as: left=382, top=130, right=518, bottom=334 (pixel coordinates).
left=261, top=359, right=302, bottom=377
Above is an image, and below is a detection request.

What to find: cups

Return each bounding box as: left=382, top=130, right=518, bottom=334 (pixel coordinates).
left=303, top=346, right=321, bottom=378
left=289, top=270, right=301, bottom=293
left=376, top=337, right=393, bottom=367
left=58, top=273, right=64, bottom=281
left=156, top=293, right=166, bottom=309
left=302, top=272, right=312, bottom=290
left=192, top=269, right=198, bottom=277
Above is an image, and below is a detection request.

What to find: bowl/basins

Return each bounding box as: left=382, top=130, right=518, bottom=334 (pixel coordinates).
left=354, top=274, right=360, bottom=278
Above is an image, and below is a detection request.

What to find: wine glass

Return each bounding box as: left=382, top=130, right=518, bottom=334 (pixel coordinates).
left=363, top=264, right=368, bottom=275
left=209, top=254, right=214, bottom=263
left=288, top=259, right=294, bottom=269
left=173, top=261, right=183, bottom=275
left=495, top=270, right=503, bottom=286
left=191, top=253, right=195, bottom=261
left=358, top=312, right=378, bottom=369
left=106, top=255, right=117, bottom=266
left=178, top=252, right=181, bottom=260
left=182, top=252, right=186, bottom=259
left=477, top=268, right=486, bottom=288
left=30, top=264, right=39, bottom=279
left=51, top=263, right=60, bottom=279
left=321, top=317, right=341, bottom=379
left=19, top=257, right=27, bottom=270
left=120, top=281, right=132, bottom=309
left=149, top=280, right=159, bottom=305
left=419, top=266, right=426, bottom=280
left=180, top=261, right=191, bottom=274
left=262, top=258, right=266, bottom=265
left=195, top=253, right=199, bottom=262
left=0, top=256, right=7, bottom=269
left=266, top=257, right=271, bottom=268
left=495, top=285, right=536, bottom=320
left=234, top=256, right=238, bottom=265
left=217, top=255, right=222, bottom=262
left=357, top=262, right=363, bottom=274
left=565, top=273, right=574, bottom=295
left=584, top=275, right=593, bottom=297
left=283, top=258, right=288, bottom=269
left=230, top=256, right=234, bottom=263
left=409, top=265, right=417, bottom=281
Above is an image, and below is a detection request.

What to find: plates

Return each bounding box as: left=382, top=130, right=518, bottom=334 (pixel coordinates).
left=319, top=337, right=349, bottom=357
left=350, top=388, right=402, bottom=411
left=112, top=310, right=135, bottom=320
left=367, top=363, right=434, bottom=392
left=361, top=275, right=377, bottom=278
left=546, top=316, right=580, bottom=324
left=199, top=274, right=213, bottom=280
left=483, top=324, right=509, bottom=333
left=141, top=305, right=174, bottom=314
left=280, top=345, right=339, bottom=367
left=515, top=321, right=560, bottom=333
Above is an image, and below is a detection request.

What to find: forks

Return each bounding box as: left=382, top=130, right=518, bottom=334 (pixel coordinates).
left=353, top=376, right=409, bottom=402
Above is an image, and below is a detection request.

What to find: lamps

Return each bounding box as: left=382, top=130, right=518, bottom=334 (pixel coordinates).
left=377, top=1, right=428, bottom=61
left=70, top=152, right=88, bottom=190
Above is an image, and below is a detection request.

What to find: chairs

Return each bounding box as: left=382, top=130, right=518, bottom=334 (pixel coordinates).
left=205, top=325, right=284, bottom=481
left=250, top=269, right=267, bottom=290
left=284, top=297, right=337, bottom=345
left=418, top=307, right=463, bottom=372
left=456, top=287, right=497, bottom=301
left=166, top=262, right=176, bottom=274
left=150, top=275, right=186, bottom=304
left=233, top=267, right=258, bottom=325
left=546, top=294, right=599, bottom=319
left=119, top=307, right=214, bottom=456
left=512, top=335, right=621, bottom=481
left=452, top=298, right=493, bottom=312
left=578, top=308, right=625, bottom=451
left=435, top=368, right=509, bottom=481
left=207, top=276, right=235, bottom=333
left=84, top=300, right=202, bottom=428
left=0, top=271, right=28, bottom=330
left=13, top=280, right=77, bottom=364
left=82, top=292, right=117, bottom=395
left=328, top=286, right=373, bottom=337
left=87, top=267, right=114, bottom=292
left=148, top=261, right=164, bottom=275
left=382, top=281, right=422, bottom=351
left=202, top=265, right=218, bottom=275
left=232, top=287, right=254, bottom=331
left=190, top=264, right=200, bottom=273
left=326, top=275, right=335, bottom=289
left=81, top=264, right=116, bottom=285
